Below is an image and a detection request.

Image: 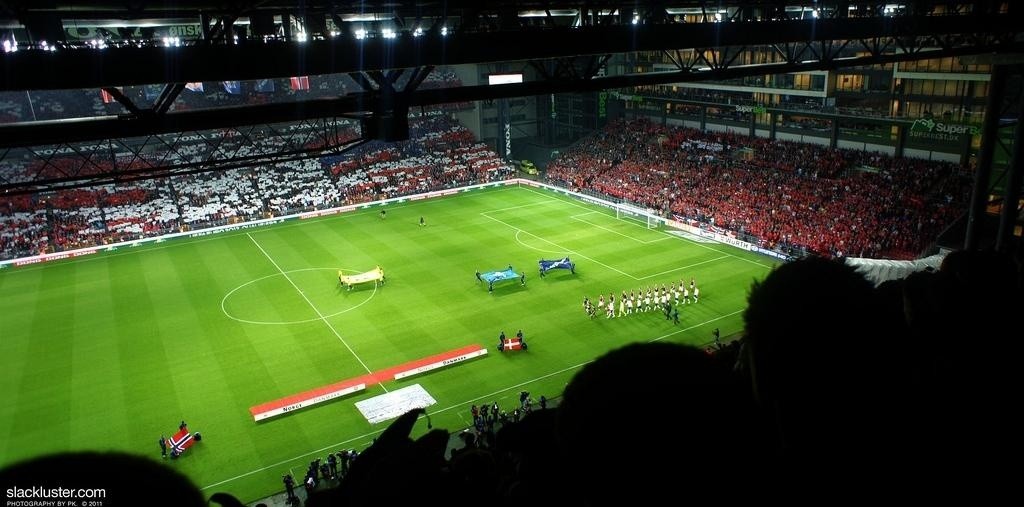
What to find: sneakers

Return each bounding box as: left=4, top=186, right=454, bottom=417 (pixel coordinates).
left=606, top=299, right=699, bottom=320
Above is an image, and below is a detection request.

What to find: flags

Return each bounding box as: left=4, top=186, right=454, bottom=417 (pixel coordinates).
left=165, top=428, right=197, bottom=456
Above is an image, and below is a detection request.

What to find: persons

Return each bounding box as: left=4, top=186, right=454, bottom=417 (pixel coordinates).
left=256, top=268, right=1024, bottom=506
left=159, top=433, right=167, bottom=459
left=170, top=447, right=179, bottom=461
left=0, top=93, right=1022, bottom=273
left=178, top=421, right=189, bottom=432
left=192, top=432, right=201, bottom=443
